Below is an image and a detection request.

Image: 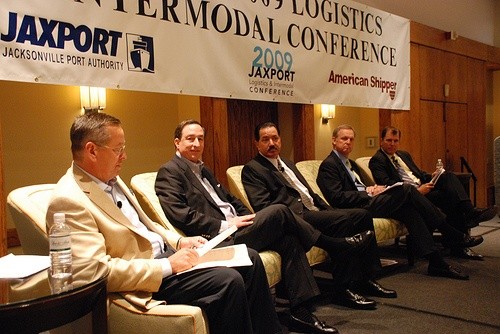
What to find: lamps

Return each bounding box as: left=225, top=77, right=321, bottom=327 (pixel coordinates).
left=321, top=104, right=336, bottom=123
left=80, top=85, right=107, bottom=111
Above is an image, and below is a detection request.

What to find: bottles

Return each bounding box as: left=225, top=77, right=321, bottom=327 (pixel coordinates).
left=436, top=159, right=443, bottom=171
left=49, top=213, right=72, bottom=278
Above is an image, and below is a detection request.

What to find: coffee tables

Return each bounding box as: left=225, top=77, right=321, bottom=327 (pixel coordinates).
left=0, top=255, right=110, bottom=334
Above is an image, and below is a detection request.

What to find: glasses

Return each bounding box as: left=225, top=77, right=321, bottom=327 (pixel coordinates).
left=102, top=146, right=125, bottom=154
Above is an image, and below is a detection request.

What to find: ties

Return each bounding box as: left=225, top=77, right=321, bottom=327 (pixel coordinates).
left=345, top=158, right=366, bottom=187
left=277, top=158, right=320, bottom=212
left=391, top=155, right=420, bottom=187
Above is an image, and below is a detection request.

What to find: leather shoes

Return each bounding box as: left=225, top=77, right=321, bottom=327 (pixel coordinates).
left=287, top=312, right=338, bottom=334
left=457, top=246, right=485, bottom=261
left=463, top=236, right=483, bottom=248
left=473, top=205, right=498, bottom=226
left=342, top=288, right=375, bottom=309
left=369, top=282, right=397, bottom=298
left=428, top=263, right=469, bottom=280
left=344, top=230, right=375, bottom=253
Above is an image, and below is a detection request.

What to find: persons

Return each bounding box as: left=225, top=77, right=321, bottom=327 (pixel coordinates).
left=46, top=112, right=286, bottom=334
left=317, top=124, right=483, bottom=279
left=368, top=124, right=497, bottom=259
left=242, top=121, right=396, bottom=310
left=155, top=120, right=375, bottom=334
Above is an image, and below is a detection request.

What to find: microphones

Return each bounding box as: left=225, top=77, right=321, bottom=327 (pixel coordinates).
left=116, top=201, right=123, bottom=208
left=350, top=167, right=354, bottom=171
left=281, top=167, right=284, bottom=171
left=394, top=160, right=398, bottom=163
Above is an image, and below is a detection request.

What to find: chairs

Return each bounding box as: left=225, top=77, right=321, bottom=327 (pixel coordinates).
left=130, top=172, right=280, bottom=289
left=294, top=159, right=400, bottom=246
left=226, top=166, right=327, bottom=266
left=354, top=157, right=447, bottom=237
left=7, top=183, right=208, bottom=334
